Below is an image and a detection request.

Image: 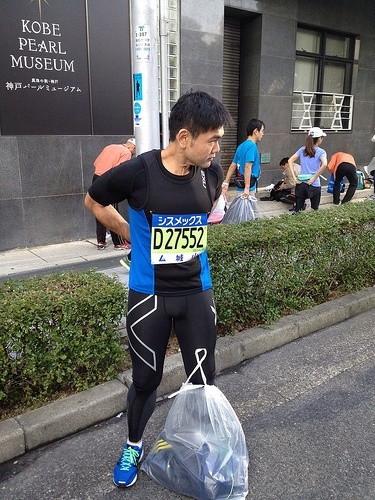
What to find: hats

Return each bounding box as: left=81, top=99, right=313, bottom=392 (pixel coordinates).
left=128, top=138, right=135, bottom=145
left=308, top=127, right=327, bottom=138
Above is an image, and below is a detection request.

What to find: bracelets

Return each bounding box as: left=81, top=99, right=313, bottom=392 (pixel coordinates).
left=222, top=182, right=229, bottom=186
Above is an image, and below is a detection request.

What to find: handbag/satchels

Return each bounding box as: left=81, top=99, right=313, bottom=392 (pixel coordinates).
left=235, top=175, right=256, bottom=188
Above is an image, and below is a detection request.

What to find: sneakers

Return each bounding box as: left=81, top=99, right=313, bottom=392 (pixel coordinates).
left=114, top=241, right=131, bottom=249
left=120, top=256, right=131, bottom=270
left=112, top=438, right=144, bottom=489
left=98, top=242, right=108, bottom=250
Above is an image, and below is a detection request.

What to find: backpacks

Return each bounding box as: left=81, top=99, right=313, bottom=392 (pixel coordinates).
left=327, top=174, right=345, bottom=193
left=356, top=169, right=365, bottom=190
left=270, top=181, right=291, bottom=200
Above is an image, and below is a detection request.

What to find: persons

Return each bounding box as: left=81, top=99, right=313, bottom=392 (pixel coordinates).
left=290, top=126, right=326, bottom=209
left=329, top=149, right=356, bottom=202
left=223, top=117, right=266, bottom=199
left=277, top=157, right=302, bottom=194
left=85, top=88, right=222, bottom=488
left=90, top=136, right=135, bottom=249
